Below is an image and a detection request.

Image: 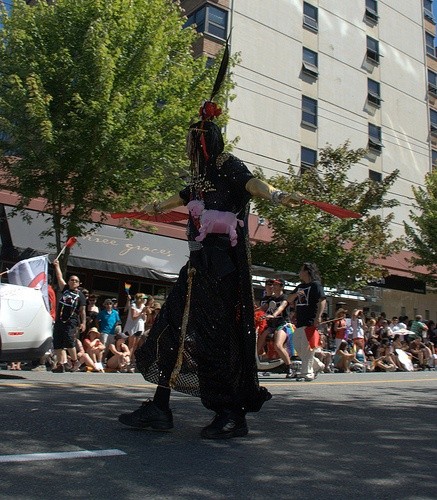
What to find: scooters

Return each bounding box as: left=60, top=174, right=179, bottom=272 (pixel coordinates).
left=253, top=300, right=326, bottom=381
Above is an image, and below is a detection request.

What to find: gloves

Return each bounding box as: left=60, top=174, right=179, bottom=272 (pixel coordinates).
left=282, top=190, right=305, bottom=208
left=144, top=200, right=163, bottom=217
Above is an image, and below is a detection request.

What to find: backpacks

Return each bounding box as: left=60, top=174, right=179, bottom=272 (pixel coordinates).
left=330, top=320, right=341, bottom=340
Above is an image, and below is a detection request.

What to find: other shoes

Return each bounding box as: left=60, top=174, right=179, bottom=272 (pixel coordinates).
left=94, top=362, right=103, bottom=369
left=65, top=363, right=72, bottom=371
left=120, top=364, right=132, bottom=372
left=314, top=364, right=325, bottom=379
left=296, top=377, right=311, bottom=382
left=286, top=367, right=294, bottom=378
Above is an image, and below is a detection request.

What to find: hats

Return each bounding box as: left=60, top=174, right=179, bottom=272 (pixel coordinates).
left=141, top=294, right=148, bottom=299
left=103, top=299, right=114, bottom=306
left=88, top=327, right=100, bottom=336
left=153, top=303, right=161, bottom=309
left=273, top=280, right=285, bottom=287
left=335, top=308, right=348, bottom=316
left=116, top=333, right=129, bottom=339
left=353, top=309, right=358, bottom=315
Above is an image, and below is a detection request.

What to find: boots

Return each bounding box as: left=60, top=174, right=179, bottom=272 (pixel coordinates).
left=51, top=363, right=64, bottom=373
left=69, top=359, right=81, bottom=372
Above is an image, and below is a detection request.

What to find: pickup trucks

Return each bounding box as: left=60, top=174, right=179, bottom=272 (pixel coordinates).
left=0, top=281, right=58, bottom=366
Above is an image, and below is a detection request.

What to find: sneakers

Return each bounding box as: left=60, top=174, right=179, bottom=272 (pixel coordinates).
left=201, top=412, right=248, bottom=441
left=118, top=398, right=174, bottom=429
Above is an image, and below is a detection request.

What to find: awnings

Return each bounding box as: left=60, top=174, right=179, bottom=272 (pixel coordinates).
left=5, top=206, right=190, bottom=282
left=0, top=154, right=437, bottom=284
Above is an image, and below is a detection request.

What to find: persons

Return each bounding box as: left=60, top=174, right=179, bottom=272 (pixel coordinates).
left=118, top=121, right=314, bottom=441
left=255, top=278, right=297, bottom=379
left=47, top=258, right=162, bottom=373
left=288, top=308, right=437, bottom=374
left=10, top=362, right=21, bottom=370
left=287, top=262, right=327, bottom=382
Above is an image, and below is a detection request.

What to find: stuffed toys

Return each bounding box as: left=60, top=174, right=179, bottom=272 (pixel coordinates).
left=187, top=200, right=244, bottom=247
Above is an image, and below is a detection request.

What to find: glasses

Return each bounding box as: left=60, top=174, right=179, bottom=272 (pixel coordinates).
left=69, top=279, right=79, bottom=282
left=89, top=299, right=96, bottom=301
left=267, top=284, right=273, bottom=286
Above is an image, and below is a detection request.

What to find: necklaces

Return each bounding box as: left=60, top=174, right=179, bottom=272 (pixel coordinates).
left=60, top=289, right=79, bottom=323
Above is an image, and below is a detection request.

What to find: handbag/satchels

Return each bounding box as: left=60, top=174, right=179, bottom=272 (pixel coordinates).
left=185, top=199, right=244, bottom=247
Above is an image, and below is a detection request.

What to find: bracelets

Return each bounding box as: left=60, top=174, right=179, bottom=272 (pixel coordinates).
left=272, top=190, right=288, bottom=203
